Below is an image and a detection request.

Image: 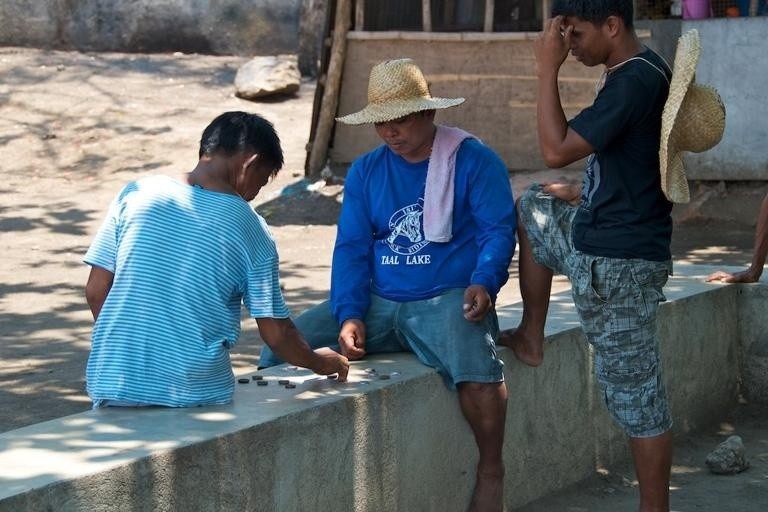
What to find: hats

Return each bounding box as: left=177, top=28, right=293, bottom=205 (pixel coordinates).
left=658, top=28, right=727, bottom=206
left=333, top=57, right=466, bottom=128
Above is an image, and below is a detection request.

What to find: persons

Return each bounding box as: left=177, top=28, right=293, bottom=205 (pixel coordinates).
left=492, top=1, right=678, bottom=512
left=80, top=110, right=351, bottom=412
left=700, top=189, right=768, bottom=288
left=254, top=56, right=520, bottom=512
